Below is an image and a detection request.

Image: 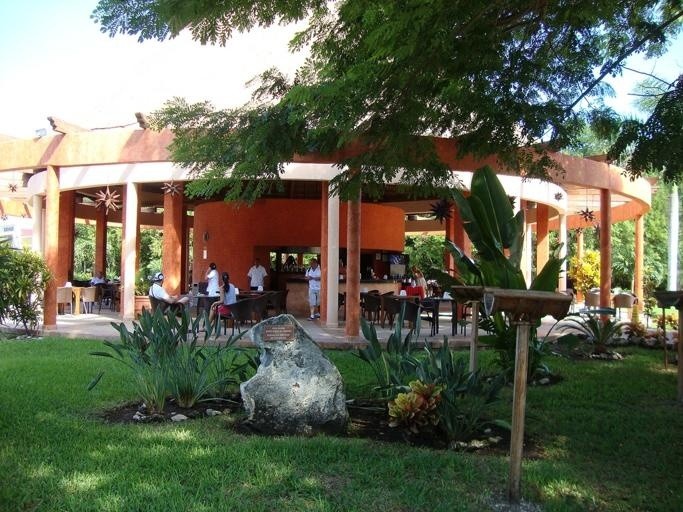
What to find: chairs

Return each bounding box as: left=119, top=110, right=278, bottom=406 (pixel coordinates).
left=360, top=287, right=469, bottom=334
left=147, top=283, right=290, bottom=335
left=584, top=287, right=638, bottom=321
left=57, top=281, right=120, bottom=314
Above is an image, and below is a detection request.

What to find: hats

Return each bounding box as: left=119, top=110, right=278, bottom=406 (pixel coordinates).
left=151, top=271, right=164, bottom=281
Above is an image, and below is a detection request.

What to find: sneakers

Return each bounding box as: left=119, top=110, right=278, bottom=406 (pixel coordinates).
left=307, top=312, right=320, bottom=321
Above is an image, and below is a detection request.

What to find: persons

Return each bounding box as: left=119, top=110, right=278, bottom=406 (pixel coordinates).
left=148, top=271, right=192, bottom=313
left=203, top=262, right=220, bottom=296
left=303, top=258, right=321, bottom=321
left=283, top=256, right=295, bottom=273
left=207, top=272, right=236, bottom=328
left=246, top=257, right=268, bottom=291
left=411, top=264, right=426, bottom=294
left=89, top=271, right=108, bottom=285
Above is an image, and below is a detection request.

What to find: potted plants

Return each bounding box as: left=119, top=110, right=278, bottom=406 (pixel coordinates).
left=134, top=288, right=152, bottom=319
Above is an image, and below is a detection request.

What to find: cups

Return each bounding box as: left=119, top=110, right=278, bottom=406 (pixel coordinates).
left=400, top=290, right=406, bottom=296
left=235, top=288, right=239, bottom=295
left=258, top=286, right=263, bottom=291
left=66, top=282, right=72, bottom=287
